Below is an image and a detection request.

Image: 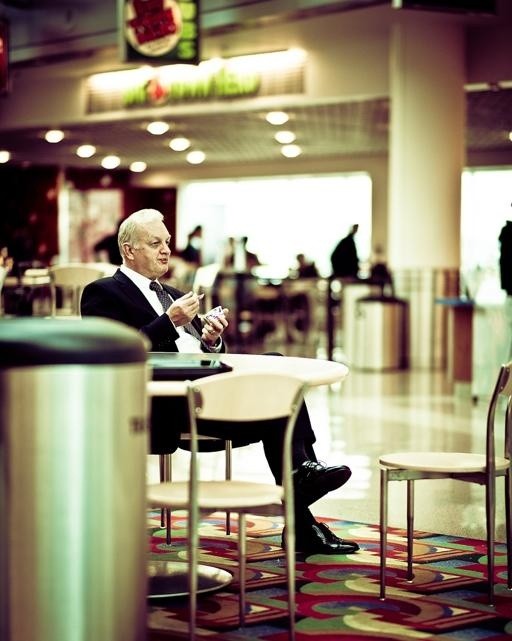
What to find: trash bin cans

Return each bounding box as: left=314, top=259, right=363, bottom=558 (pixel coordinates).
left=352, top=296, right=405, bottom=370
left=0, top=317, right=150, bottom=641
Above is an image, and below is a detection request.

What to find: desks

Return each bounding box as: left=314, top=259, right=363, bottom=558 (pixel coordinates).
left=146, top=353, right=350, bottom=603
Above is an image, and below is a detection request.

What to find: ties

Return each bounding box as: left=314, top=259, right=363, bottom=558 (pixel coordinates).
left=148, top=280, right=214, bottom=353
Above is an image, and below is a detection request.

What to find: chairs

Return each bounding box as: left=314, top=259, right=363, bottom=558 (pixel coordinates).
left=41, top=266, right=103, bottom=320
left=145, top=372, right=308, bottom=641
left=379, top=362, right=512, bottom=608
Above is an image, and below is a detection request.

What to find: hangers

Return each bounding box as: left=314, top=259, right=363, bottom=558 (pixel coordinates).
left=141, top=504, right=512, bottom=641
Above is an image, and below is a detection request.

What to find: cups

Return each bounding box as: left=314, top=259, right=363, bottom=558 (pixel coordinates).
left=202, top=306, right=226, bottom=330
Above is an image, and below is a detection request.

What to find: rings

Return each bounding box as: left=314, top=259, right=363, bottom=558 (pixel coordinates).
left=211, top=331, right=215, bottom=335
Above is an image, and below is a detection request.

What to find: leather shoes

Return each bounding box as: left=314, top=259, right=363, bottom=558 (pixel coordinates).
left=279, top=519, right=360, bottom=554
left=293, top=459, right=352, bottom=510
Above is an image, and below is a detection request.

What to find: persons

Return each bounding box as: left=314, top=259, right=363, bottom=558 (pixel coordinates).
left=241, top=236, right=264, bottom=273
left=182, top=224, right=203, bottom=262
left=78, top=208, right=361, bottom=557
left=223, top=237, right=237, bottom=267
left=94, top=217, right=128, bottom=267
left=367, top=242, right=391, bottom=284
left=330, top=224, right=360, bottom=279
left=296, top=254, right=321, bottom=278
